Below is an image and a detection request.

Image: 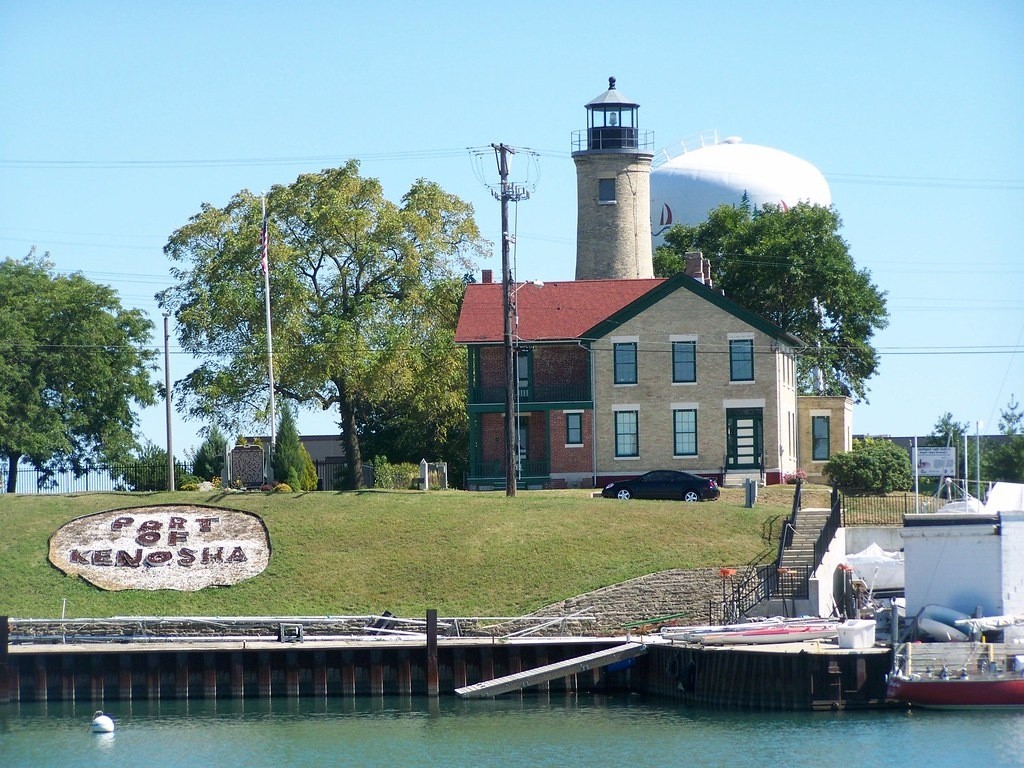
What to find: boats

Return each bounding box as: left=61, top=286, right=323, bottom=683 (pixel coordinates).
left=886, top=661, right=1024, bottom=709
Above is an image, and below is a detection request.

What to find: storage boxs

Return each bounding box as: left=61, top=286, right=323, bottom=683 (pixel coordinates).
left=836, top=618, right=876, bottom=649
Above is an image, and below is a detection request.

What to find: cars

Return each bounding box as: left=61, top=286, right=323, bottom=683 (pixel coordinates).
left=602, top=470, right=720, bottom=502
